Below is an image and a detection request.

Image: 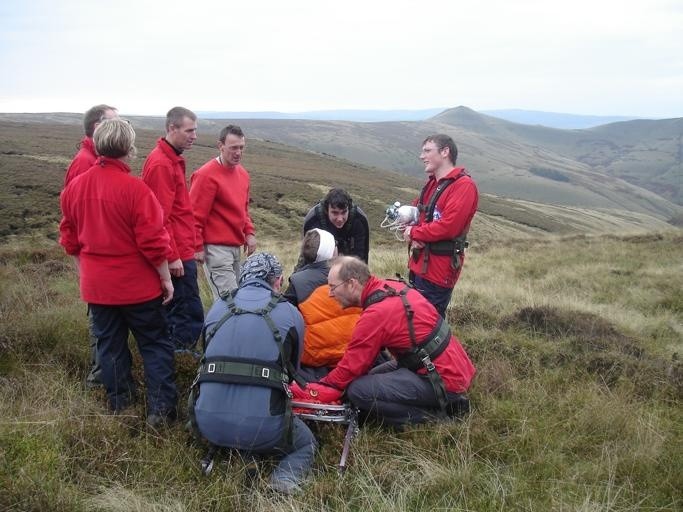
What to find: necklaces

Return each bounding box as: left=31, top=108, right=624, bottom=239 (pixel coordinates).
left=217, top=155, right=224, bottom=167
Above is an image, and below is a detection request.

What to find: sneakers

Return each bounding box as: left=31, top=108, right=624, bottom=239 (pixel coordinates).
left=148, top=415, right=172, bottom=428
left=271, top=478, right=304, bottom=497
left=205, top=455, right=227, bottom=479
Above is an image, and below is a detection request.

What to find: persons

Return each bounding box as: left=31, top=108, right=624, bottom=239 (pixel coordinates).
left=182, top=251, right=322, bottom=496
left=141, top=106, right=205, bottom=362
left=61, top=105, right=144, bottom=406
left=317, top=254, right=477, bottom=432
left=184, top=124, right=258, bottom=303
left=302, top=187, right=369, bottom=268
left=277, top=227, right=386, bottom=384
left=397, top=134, right=478, bottom=325
left=56, top=118, right=182, bottom=438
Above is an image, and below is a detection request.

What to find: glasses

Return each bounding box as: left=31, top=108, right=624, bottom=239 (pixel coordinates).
left=328, top=279, right=348, bottom=292
